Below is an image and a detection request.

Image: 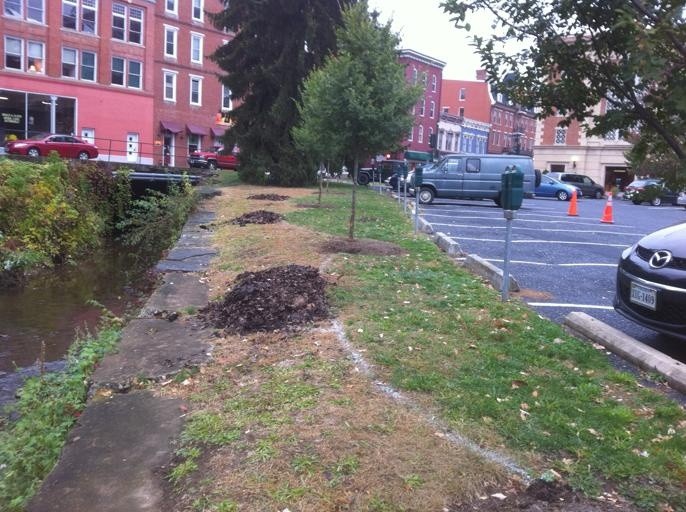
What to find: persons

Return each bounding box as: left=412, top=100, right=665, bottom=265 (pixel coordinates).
left=164, top=145, right=171, bottom=166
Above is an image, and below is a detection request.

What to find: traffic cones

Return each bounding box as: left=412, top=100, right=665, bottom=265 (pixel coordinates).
left=601, top=192, right=615, bottom=224
left=568, top=187, right=580, bottom=219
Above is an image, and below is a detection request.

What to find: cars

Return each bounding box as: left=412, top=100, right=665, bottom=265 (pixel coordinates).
left=625, top=180, right=685, bottom=205
left=613, top=219, right=686, bottom=342
left=185, top=142, right=242, bottom=172
left=317, top=153, right=605, bottom=207
left=5, top=126, right=99, bottom=164
